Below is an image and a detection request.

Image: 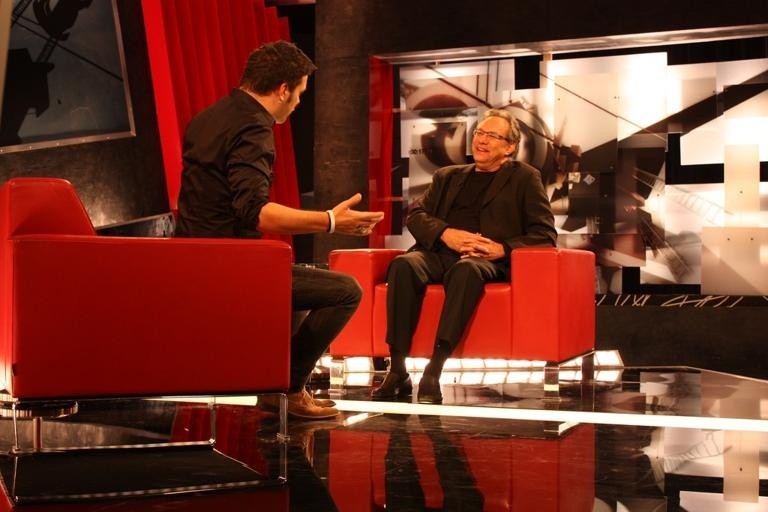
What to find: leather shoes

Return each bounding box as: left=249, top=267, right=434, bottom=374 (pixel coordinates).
left=310, top=398, right=336, bottom=408
left=257, top=389, right=342, bottom=419
left=416, top=374, right=442, bottom=404
left=370, top=373, right=413, bottom=399
left=256, top=419, right=346, bottom=447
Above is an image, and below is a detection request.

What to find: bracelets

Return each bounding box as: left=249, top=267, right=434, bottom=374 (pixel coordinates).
left=327, top=209, right=336, bottom=234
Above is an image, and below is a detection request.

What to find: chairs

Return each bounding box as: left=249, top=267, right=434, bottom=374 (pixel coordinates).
left=325, top=244, right=598, bottom=440
left=0, top=178, right=295, bottom=512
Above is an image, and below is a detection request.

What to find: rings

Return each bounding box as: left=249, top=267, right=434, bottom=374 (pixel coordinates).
left=359, top=226, right=367, bottom=235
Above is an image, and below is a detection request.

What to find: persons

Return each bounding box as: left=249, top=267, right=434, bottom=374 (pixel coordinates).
left=375, top=408, right=486, bottom=511
left=257, top=416, right=344, bottom=510
left=170, top=37, right=389, bottom=419
left=369, top=107, right=557, bottom=404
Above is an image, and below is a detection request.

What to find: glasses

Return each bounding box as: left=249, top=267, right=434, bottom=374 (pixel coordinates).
left=473, top=127, right=510, bottom=143
